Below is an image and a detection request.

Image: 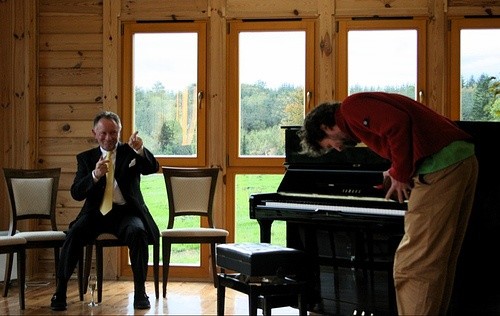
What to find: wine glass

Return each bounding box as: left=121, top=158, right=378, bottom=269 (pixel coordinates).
left=87, top=274, right=99, bottom=305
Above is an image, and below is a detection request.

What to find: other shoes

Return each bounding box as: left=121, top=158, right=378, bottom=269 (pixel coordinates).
left=134, top=293, right=150, bottom=309
left=50, top=293, right=67, bottom=311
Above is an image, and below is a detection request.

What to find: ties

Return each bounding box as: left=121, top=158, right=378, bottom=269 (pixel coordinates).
left=98, top=151, right=115, bottom=217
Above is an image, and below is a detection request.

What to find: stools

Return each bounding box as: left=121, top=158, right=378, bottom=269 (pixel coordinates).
left=216, top=241, right=307, bottom=316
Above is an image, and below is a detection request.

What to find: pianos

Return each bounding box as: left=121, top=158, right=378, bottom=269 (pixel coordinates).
left=247, top=119, right=500, bottom=316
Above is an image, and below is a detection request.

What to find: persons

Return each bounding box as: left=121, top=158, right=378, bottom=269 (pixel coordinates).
left=303, top=92, right=478, bottom=316
left=50, top=110, right=159, bottom=311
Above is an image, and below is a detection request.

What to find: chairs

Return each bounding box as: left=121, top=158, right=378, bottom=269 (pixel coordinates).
left=0, top=166, right=229, bottom=311
